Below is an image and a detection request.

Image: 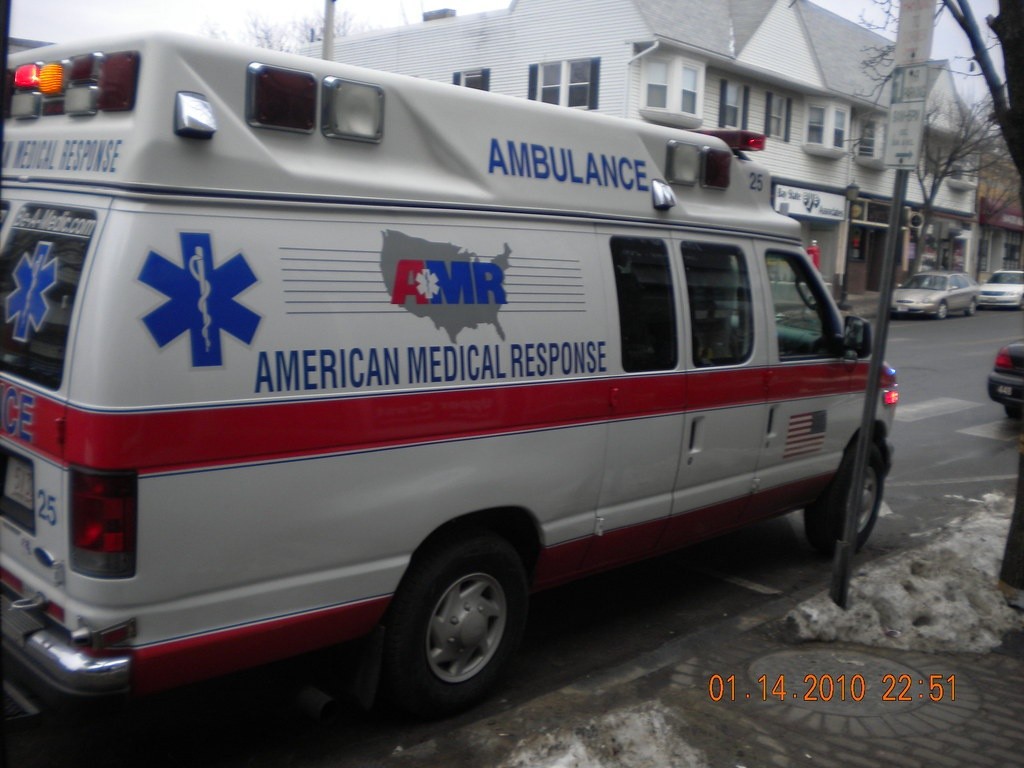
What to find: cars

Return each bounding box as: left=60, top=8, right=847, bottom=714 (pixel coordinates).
left=890, top=270, right=980, bottom=320
left=986, top=342, right=1024, bottom=419
left=978, top=270, right=1024, bottom=311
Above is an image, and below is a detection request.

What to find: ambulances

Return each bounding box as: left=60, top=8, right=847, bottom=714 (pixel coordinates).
left=0, top=31, right=899, bottom=720
